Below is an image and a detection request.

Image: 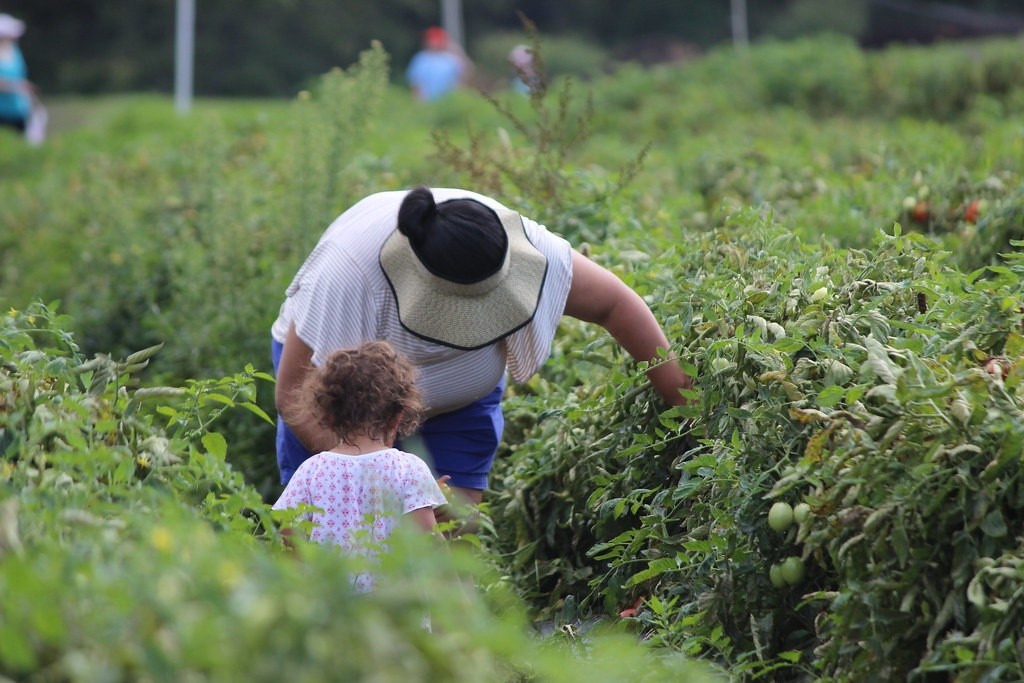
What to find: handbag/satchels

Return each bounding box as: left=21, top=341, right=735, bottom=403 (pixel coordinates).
left=23, top=96, right=49, bottom=148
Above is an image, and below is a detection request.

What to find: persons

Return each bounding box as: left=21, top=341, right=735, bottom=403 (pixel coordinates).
left=0, top=13, right=48, bottom=146
left=271, top=187, right=698, bottom=550
left=271, top=341, right=472, bottom=633
left=404, top=27, right=476, bottom=109
left=506, top=43, right=547, bottom=97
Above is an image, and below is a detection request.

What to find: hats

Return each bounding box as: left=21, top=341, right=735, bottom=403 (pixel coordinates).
left=424, top=27, right=446, bottom=47
left=1, top=13, right=27, bottom=37
left=380, top=189, right=549, bottom=349
